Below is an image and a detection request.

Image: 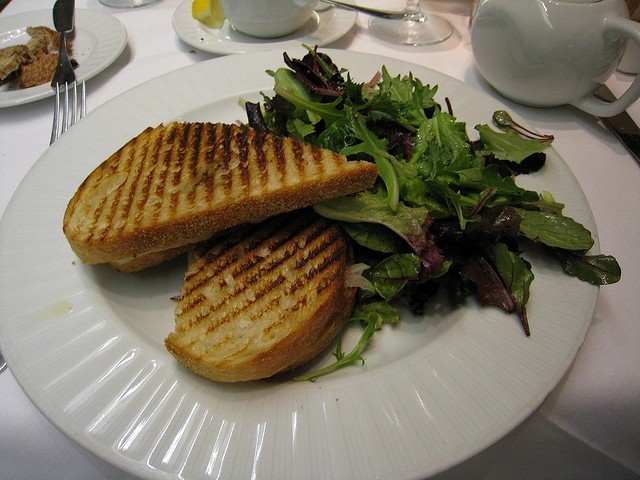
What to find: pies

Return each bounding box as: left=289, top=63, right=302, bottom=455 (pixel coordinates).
left=62, top=120, right=377, bottom=385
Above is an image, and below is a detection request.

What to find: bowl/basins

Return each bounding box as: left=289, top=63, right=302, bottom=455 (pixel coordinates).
left=220, top=0, right=319, bottom=39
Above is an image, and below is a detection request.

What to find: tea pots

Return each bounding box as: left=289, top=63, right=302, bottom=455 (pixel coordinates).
left=470, top=0, right=640, bottom=120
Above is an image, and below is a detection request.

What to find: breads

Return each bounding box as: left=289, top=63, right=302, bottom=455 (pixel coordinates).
left=2, top=17, right=62, bottom=90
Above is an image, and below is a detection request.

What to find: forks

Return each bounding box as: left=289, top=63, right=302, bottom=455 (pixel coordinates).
left=49, top=80, right=86, bottom=147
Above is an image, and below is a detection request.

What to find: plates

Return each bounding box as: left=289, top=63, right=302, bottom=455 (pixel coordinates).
left=1, top=1, right=130, bottom=109
left=172, top=0, right=358, bottom=54
left=0, top=50, right=601, bottom=479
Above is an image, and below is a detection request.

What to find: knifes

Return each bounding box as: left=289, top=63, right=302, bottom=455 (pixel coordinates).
left=49, top=0, right=75, bottom=88
left=593, top=82, right=639, bottom=164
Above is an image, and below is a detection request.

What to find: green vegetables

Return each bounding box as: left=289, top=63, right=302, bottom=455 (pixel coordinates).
left=244, top=40, right=622, bottom=386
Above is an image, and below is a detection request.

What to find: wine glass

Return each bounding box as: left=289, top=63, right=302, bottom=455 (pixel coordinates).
left=367, top=0, right=455, bottom=48
